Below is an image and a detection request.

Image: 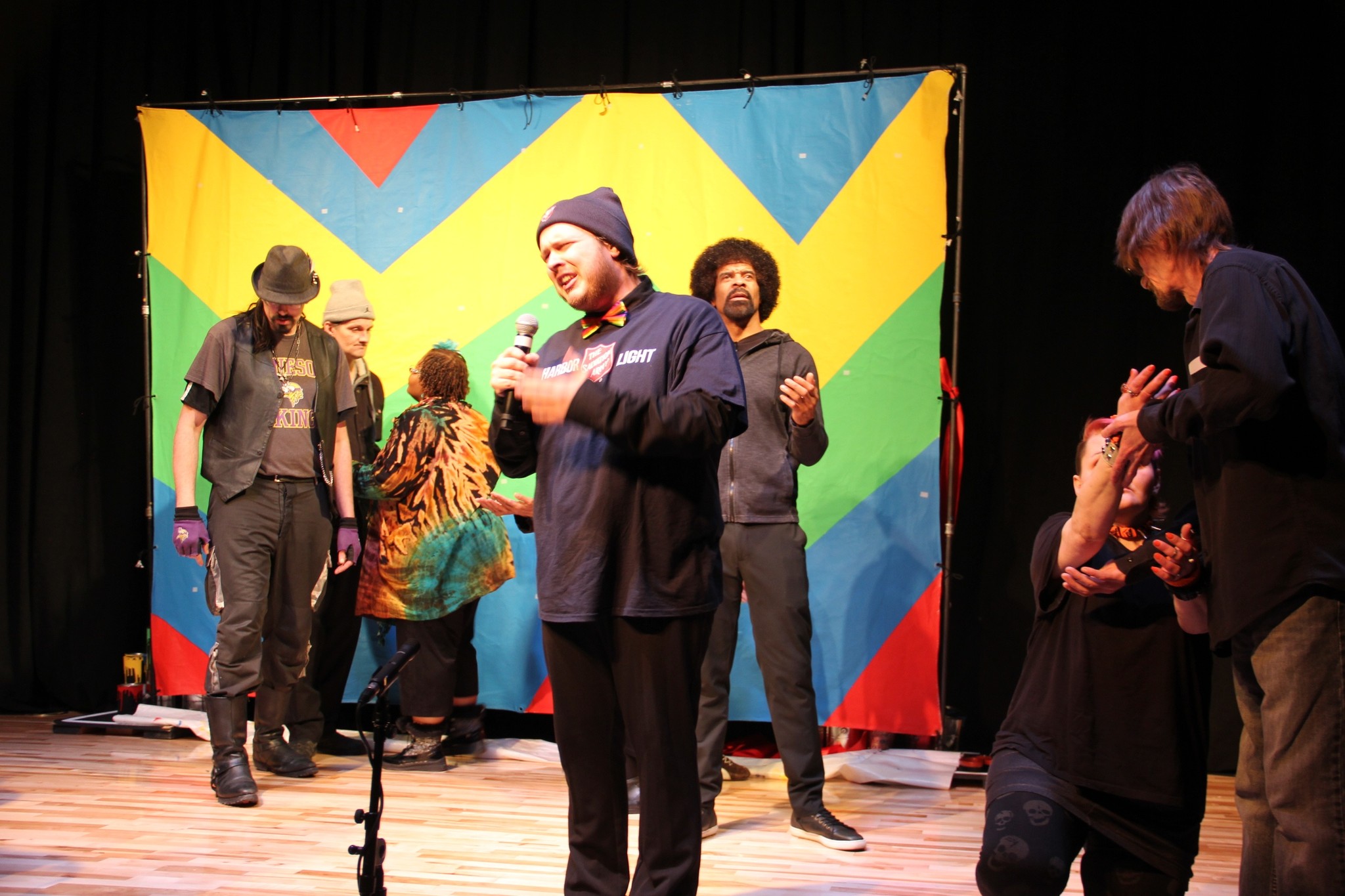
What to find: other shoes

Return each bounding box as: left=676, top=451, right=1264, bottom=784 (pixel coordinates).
left=380, top=741, right=449, bottom=772
left=287, top=738, right=318, bottom=761
left=316, top=731, right=367, bottom=755
left=439, top=719, right=486, bottom=759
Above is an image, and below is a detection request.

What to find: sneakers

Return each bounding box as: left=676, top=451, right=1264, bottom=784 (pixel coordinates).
left=790, top=806, right=866, bottom=851
left=701, top=805, right=719, bottom=839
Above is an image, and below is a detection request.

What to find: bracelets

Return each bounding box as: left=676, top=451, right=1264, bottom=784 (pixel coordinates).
left=1101, top=437, right=1119, bottom=466
left=1163, top=567, right=1206, bottom=601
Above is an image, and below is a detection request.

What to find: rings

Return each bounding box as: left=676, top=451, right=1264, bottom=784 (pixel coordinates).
left=1121, top=384, right=1138, bottom=396
left=1181, top=529, right=1196, bottom=546
left=1171, top=548, right=1177, bottom=558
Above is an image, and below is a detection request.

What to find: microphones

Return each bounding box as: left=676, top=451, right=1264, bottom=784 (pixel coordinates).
left=358, top=638, right=422, bottom=705
left=501, top=314, right=539, bottom=431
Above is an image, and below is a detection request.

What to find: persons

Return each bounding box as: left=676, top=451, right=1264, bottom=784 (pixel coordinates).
left=691, top=238, right=867, bottom=852
left=172, top=244, right=362, bottom=805
left=490, top=186, right=748, bottom=896
left=290, top=279, right=516, bottom=771
left=976, top=366, right=1213, bottom=896
left=1102, top=167, right=1345, bottom=896
left=474, top=491, right=641, bottom=811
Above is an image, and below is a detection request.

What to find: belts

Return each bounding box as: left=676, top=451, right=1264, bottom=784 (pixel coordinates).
left=255, top=473, right=314, bottom=484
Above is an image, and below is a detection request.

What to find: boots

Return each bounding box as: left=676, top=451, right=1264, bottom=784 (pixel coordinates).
left=253, top=684, right=319, bottom=778
left=201, top=695, right=259, bottom=806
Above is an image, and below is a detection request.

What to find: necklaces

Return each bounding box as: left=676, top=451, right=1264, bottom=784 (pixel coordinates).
left=273, top=321, right=303, bottom=391
left=1111, top=520, right=1153, bottom=539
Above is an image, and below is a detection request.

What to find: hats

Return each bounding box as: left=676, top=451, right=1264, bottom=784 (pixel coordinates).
left=536, top=187, right=637, bottom=267
left=322, top=278, right=375, bottom=326
left=251, top=245, right=320, bottom=304
